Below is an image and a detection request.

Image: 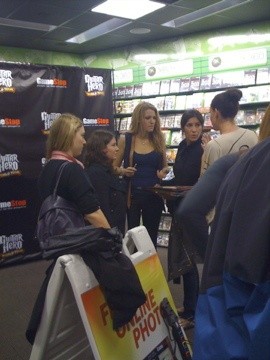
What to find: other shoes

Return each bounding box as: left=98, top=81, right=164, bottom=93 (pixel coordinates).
left=176, top=308, right=195, bottom=329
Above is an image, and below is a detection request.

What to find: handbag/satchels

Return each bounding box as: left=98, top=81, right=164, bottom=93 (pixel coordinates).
left=125, top=183, right=132, bottom=209
left=40, top=225, right=124, bottom=258
left=36, top=162, right=86, bottom=259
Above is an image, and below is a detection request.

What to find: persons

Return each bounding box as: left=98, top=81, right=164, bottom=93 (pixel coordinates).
left=199, top=90, right=257, bottom=228
left=154, top=110, right=212, bottom=330
left=38, top=113, right=111, bottom=228
left=175, top=106, right=270, bottom=360
left=80, top=130, right=126, bottom=238
left=113, top=102, right=170, bottom=255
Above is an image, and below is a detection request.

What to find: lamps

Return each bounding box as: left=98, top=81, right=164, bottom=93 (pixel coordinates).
left=87, top=0, right=168, bottom=22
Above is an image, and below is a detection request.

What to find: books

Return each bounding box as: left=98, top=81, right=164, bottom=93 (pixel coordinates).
left=112, top=68, right=270, bottom=247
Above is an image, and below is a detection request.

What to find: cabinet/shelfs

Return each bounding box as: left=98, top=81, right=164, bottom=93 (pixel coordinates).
left=114, top=82, right=270, bottom=248
